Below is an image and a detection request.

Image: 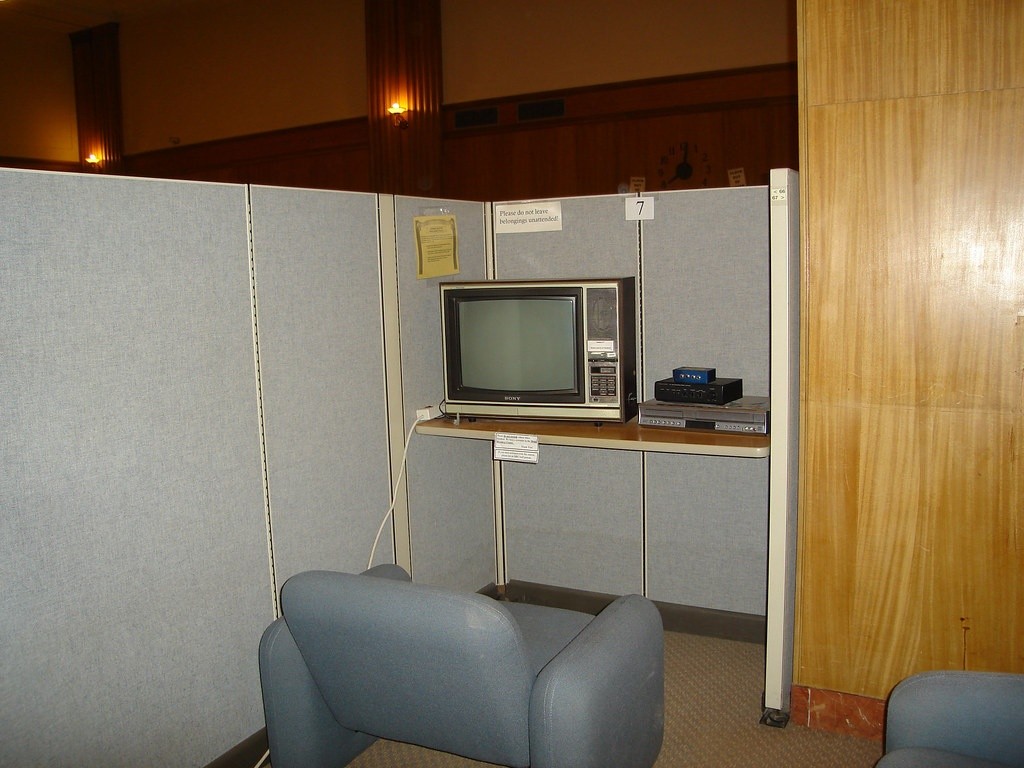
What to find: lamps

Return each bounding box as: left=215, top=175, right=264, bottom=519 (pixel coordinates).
left=387, top=103, right=408, bottom=129
left=85, top=155, right=103, bottom=174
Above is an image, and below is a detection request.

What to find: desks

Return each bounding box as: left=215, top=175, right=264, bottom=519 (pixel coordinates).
left=415, top=414, right=769, bottom=457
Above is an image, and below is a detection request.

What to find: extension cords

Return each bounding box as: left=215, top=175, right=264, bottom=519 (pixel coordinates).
left=417, top=403, right=445, bottom=421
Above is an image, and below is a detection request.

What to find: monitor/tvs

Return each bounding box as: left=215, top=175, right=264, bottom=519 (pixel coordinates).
left=439, top=276, right=638, bottom=427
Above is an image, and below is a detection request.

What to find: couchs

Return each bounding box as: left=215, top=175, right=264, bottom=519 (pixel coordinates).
left=258, top=564, right=665, bottom=768
left=876, top=669, right=1024, bottom=768
